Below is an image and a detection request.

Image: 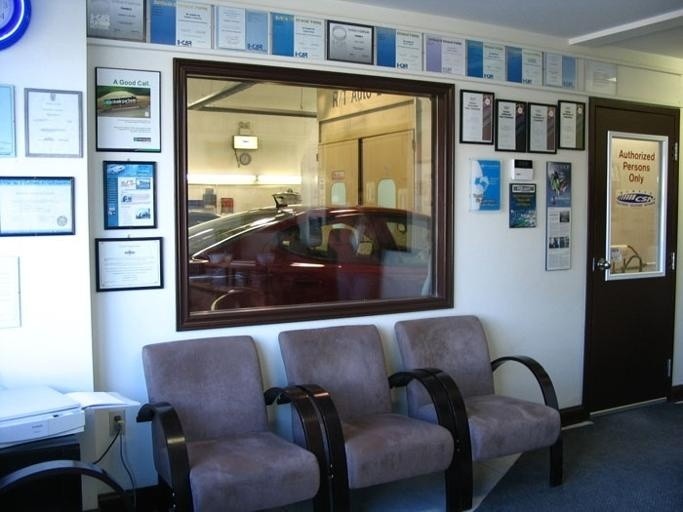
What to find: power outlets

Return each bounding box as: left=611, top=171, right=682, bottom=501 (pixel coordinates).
left=108, top=410, right=126, bottom=436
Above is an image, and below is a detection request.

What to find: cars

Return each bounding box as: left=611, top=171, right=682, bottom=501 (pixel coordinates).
left=188, top=205, right=435, bottom=314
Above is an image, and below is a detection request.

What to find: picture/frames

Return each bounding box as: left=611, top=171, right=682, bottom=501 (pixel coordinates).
left=528, top=102, right=557, bottom=154
left=327, top=19, right=374, bottom=65
left=94, top=66, right=161, bottom=153
left=557, top=100, right=585, bottom=150
left=494, top=98, right=527, bottom=153
left=94, top=237, right=164, bottom=292
left=0, top=83, right=16, bottom=158
left=0, top=176, right=76, bottom=237
left=459, top=89, right=494, bottom=145
left=102, top=160, right=157, bottom=230
left=24, top=88, right=83, bottom=158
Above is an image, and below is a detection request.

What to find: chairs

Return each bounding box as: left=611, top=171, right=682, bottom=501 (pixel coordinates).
left=394, top=314, right=563, bottom=509
left=136, top=336, right=334, bottom=512
left=278, top=323, right=460, bottom=512
